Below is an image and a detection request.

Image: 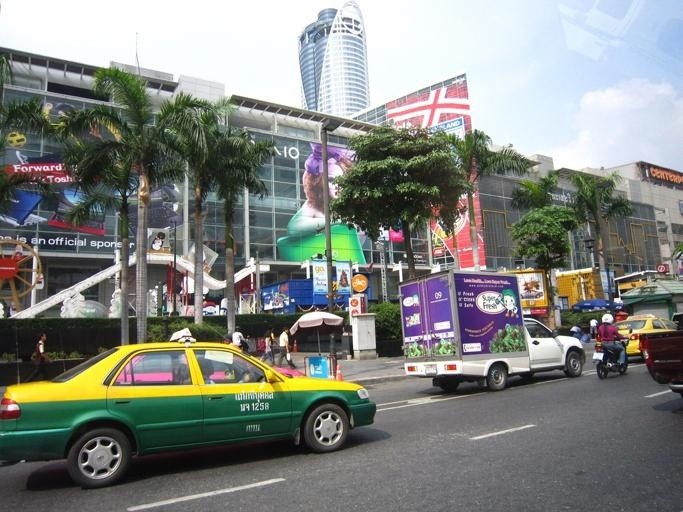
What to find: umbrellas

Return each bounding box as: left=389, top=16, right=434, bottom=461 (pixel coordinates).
left=289, top=311, right=343, bottom=356
left=572, top=299, right=623, bottom=319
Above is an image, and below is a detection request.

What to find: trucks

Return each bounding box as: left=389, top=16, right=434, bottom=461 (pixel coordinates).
left=636, top=329, right=682, bottom=398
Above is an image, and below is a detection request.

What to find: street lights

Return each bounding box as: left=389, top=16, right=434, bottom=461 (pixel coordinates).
left=317, top=115, right=344, bottom=380
left=582, top=233, right=612, bottom=312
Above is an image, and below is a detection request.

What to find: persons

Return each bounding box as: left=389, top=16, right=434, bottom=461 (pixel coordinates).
left=569, top=324, right=581, bottom=337
left=286, top=144, right=368, bottom=247
left=23, top=331, right=53, bottom=381
left=198, top=356, right=252, bottom=383
left=597, top=313, right=628, bottom=368
left=589, top=317, right=598, bottom=338
left=232, top=325, right=296, bottom=369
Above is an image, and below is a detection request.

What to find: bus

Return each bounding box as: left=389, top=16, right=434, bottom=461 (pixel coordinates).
left=613, top=269, right=659, bottom=293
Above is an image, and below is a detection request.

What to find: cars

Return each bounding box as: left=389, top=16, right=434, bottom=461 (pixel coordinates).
left=593, top=314, right=678, bottom=357
left=0, top=327, right=378, bottom=488
left=115, top=354, right=305, bottom=383
left=571, top=299, right=623, bottom=312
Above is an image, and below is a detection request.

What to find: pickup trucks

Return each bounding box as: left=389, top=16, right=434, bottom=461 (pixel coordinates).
left=393, top=270, right=586, bottom=390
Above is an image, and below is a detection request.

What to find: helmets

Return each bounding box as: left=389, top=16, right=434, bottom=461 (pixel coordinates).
left=601, top=313, right=613, bottom=324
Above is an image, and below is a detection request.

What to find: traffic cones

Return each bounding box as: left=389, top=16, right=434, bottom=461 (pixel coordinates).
left=334, top=364, right=344, bottom=381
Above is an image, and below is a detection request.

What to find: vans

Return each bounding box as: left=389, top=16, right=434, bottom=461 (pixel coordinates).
left=671, top=310, right=682, bottom=328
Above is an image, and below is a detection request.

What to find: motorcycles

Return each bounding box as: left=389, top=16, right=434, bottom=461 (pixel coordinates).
left=593, top=329, right=634, bottom=380
